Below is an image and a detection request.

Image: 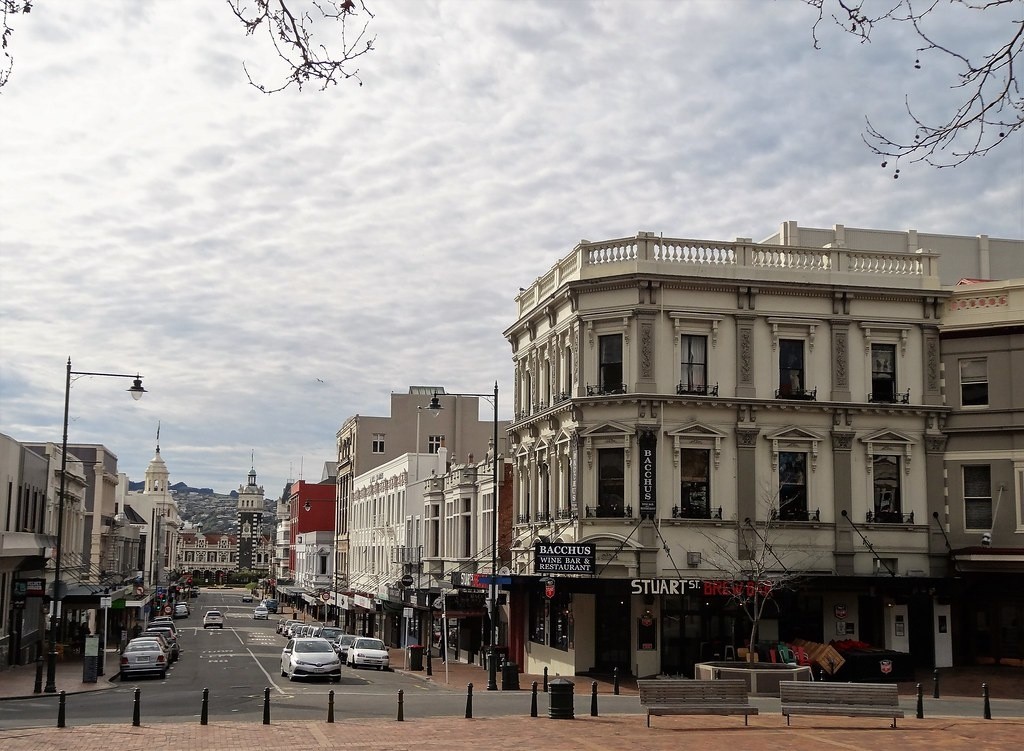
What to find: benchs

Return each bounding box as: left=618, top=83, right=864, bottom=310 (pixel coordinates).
left=778, top=680, right=905, bottom=728
left=637, top=678, right=759, bottom=728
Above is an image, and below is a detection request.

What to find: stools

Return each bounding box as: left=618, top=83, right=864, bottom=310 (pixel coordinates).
left=725, top=644, right=735, bottom=661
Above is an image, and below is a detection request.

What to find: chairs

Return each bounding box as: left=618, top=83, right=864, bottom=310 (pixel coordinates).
left=737, top=639, right=814, bottom=680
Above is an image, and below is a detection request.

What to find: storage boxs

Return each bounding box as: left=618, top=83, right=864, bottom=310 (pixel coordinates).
left=796, top=638, right=846, bottom=673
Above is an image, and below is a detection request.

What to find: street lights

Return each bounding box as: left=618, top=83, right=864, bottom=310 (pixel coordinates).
left=303, top=498, right=339, bottom=627
left=147, top=522, right=184, bottom=623
left=46, top=371, right=148, bottom=692
left=428, top=391, right=499, bottom=690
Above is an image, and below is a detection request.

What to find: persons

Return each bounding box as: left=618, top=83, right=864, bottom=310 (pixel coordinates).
left=132, top=621, right=142, bottom=639
left=115, top=621, right=123, bottom=651
left=164, top=602, right=173, bottom=615
left=79, top=622, right=91, bottom=658
left=440, top=632, right=449, bottom=664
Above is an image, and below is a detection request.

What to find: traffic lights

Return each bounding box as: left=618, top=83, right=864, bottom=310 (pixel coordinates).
left=156, top=600, right=160, bottom=611
left=162, top=594, right=166, bottom=603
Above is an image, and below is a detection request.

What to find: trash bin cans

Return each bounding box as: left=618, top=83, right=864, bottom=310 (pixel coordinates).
left=502, top=662, right=521, bottom=691
left=407, top=643, right=426, bottom=671
left=548, top=677, right=574, bottom=719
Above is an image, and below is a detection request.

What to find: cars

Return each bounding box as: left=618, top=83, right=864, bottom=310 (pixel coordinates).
left=194, top=587, right=200, bottom=593
left=131, top=617, right=179, bottom=670
left=203, top=610, right=224, bottom=629
left=173, top=602, right=191, bottom=618
left=253, top=606, right=269, bottom=620
left=280, top=636, right=342, bottom=682
left=118, top=640, right=169, bottom=679
left=262, top=600, right=278, bottom=614
left=332, top=634, right=358, bottom=661
left=347, top=636, right=390, bottom=671
left=242, top=594, right=254, bottom=603
left=188, top=590, right=197, bottom=598
left=276, top=619, right=345, bottom=648
